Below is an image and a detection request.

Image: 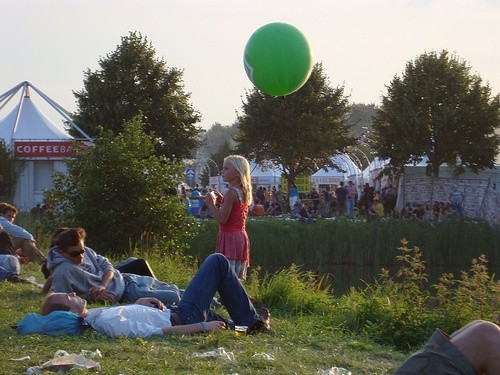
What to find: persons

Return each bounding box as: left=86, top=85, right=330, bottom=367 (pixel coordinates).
left=401, top=200, right=465, bottom=222
left=394, top=319, right=500, bottom=375
left=47, top=227, right=223, bottom=308
left=289, top=182, right=298, bottom=210
left=0, top=202, right=48, bottom=283
left=40, top=253, right=271, bottom=338
left=176, top=179, right=290, bottom=220
left=292, top=179, right=398, bottom=221
left=204, top=154, right=254, bottom=302
left=41, top=226, right=157, bottom=293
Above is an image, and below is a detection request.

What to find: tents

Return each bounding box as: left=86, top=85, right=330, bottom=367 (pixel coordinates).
left=248, top=141, right=364, bottom=186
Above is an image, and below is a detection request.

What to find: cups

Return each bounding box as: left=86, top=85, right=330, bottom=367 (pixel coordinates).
left=235, top=325, right=248, bottom=336
left=104, top=294, right=114, bottom=307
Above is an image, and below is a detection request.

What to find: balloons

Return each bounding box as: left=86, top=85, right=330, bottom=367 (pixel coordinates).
left=243, top=21, right=315, bottom=97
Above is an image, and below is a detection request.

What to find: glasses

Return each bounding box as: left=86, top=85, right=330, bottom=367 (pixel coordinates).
left=59, top=244, right=86, bottom=257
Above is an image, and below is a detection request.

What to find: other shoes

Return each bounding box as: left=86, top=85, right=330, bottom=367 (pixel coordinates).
left=261, top=307, right=271, bottom=326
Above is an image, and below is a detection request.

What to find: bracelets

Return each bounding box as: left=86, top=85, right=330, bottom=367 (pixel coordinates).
left=200, top=322, right=207, bottom=333
